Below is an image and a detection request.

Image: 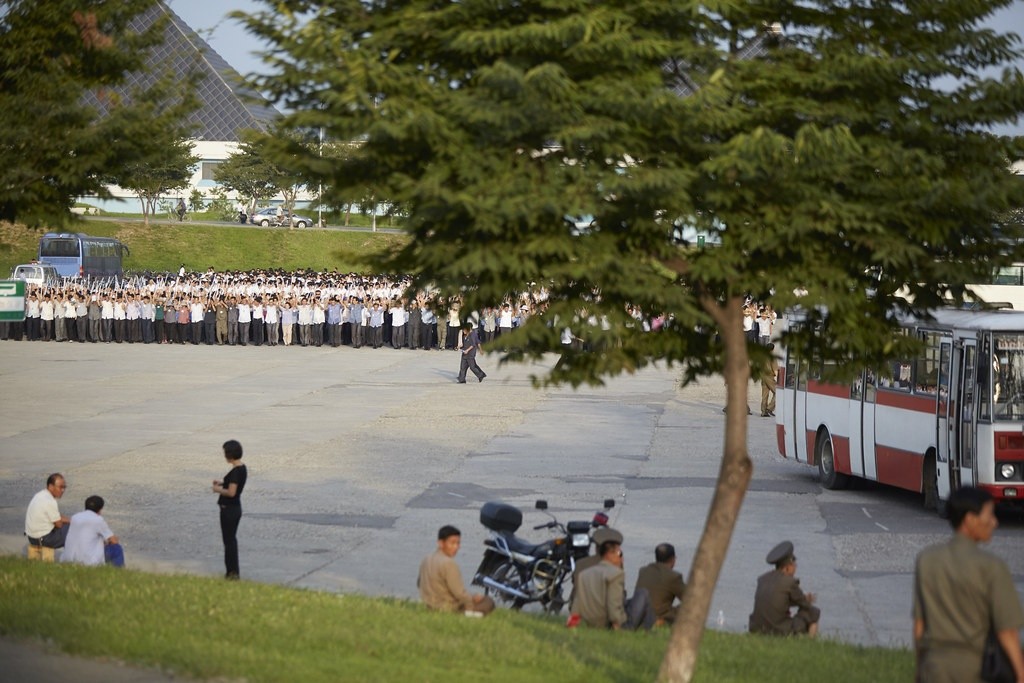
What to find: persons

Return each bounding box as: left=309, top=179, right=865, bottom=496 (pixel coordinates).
left=25, top=471, right=71, bottom=550
left=564, top=526, right=689, bottom=635
left=758, top=343, right=778, bottom=416
left=417, top=525, right=496, bottom=617
left=912, top=485, right=1023, bottom=682
left=175, top=197, right=186, bottom=221
left=212, top=440, right=248, bottom=578
left=63, top=495, right=127, bottom=570
left=750, top=542, right=819, bottom=637
left=237, top=200, right=247, bottom=224
left=24, top=268, right=824, bottom=384
left=276, top=204, right=284, bottom=224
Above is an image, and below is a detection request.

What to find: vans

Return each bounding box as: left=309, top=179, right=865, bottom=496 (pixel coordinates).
left=13, top=260, right=62, bottom=292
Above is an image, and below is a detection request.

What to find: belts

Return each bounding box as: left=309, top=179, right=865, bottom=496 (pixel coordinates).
left=28, top=532, right=51, bottom=541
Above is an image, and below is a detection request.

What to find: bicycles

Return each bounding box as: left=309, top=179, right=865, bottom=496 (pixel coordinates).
left=168, top=209, right=192, bottom=222
left=269, top=215, right=291, bottom=228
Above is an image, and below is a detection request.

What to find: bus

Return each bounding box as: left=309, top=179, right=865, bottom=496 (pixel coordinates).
left=37, top=232, right=130, bottom=285
left=774, top=303, right=1024, bottom=519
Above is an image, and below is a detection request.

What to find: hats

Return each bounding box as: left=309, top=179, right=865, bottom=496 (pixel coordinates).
left=767, top=540, right=794, bottom=566
left=592, top=527, right=623, bottom=547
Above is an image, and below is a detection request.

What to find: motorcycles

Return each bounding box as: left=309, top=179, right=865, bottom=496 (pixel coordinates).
left=471, top=500, right=615, bottom=615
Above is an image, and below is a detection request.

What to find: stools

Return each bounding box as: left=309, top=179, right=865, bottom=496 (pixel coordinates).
left=27, top=543, right=54, bottom=562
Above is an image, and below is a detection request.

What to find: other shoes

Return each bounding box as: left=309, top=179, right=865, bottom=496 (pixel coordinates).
left=457, top=381, right=467, bottom=383
left=762, top=412, right=771, bottom=417
left=26, top=336, right=458, bottom=351
left=766, top=408, right=776, bottom=416
left=479, top=374, right=486, bottom=382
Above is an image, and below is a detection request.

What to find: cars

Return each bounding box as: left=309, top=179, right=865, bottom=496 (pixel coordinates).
left=251, top=207, right=314, bottom=229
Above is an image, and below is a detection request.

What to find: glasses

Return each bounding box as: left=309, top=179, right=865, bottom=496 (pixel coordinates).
left=53, top=484, right=66, bottom=489
